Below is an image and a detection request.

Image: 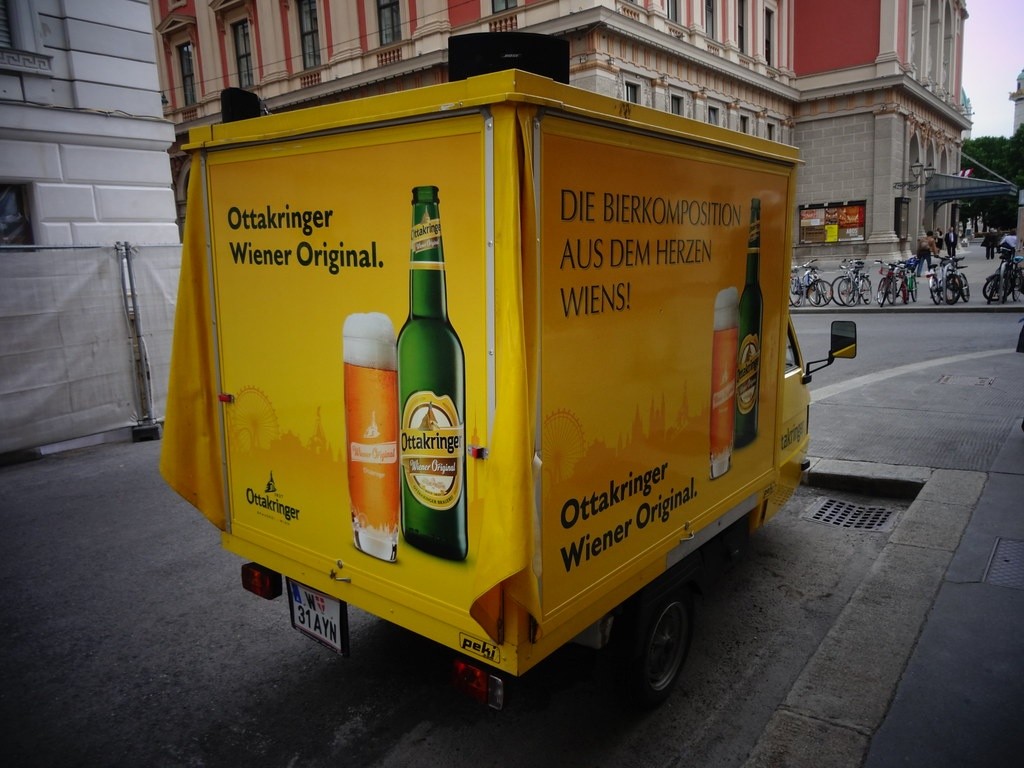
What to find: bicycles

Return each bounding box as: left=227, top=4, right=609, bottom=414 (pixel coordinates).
left=782, top=247, right=1024, bottom=306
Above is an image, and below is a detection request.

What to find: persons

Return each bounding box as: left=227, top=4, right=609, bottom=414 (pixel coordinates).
left=945, top=227, right=957, bottom=256
left=986, top=228, right=995, bottom=260
left=916, top=230, right=936, bottom=277
left=936, top=228, right=943, bottom=249
left=999, top=230, right=1016, bottom=259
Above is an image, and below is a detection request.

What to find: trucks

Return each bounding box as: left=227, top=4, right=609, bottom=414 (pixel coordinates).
left=169, top=65, right=860, bottom=714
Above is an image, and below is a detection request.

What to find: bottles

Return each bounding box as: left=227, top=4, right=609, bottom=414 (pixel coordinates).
left=734, top=194, right=764, bottom=449
left=393, top=185, right=471, bottom=562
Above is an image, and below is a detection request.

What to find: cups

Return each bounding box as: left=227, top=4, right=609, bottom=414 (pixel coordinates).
left=343, top=336, right=401, bottom=564
left=711, top=301, right=739, bottom=479
